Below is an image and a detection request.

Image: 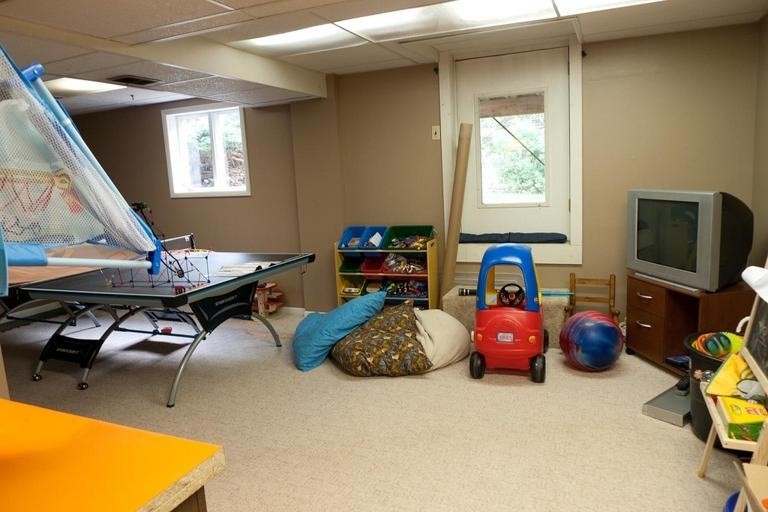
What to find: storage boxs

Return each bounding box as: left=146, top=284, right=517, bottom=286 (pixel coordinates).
left=336, top=224, right=436, bottom=282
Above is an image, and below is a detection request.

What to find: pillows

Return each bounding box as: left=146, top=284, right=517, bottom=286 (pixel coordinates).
left=412, top=306, right=472, bottom=374
left=329, top=296, right=435, bottom=378
left=291, top=289, right=389, bottom=373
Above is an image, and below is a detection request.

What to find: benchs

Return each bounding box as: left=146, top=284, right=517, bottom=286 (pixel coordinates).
left=441, top=284, right=571, bottom=350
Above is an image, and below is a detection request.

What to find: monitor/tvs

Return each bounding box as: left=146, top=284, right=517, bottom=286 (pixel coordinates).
left=626, top=189, right=754, bottom=292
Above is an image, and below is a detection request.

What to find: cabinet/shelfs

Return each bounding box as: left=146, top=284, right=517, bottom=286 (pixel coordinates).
left=332, top=232, right=441, bottom=310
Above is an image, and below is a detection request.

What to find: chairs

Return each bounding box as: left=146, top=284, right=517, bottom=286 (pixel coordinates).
left=563, top=270, right=621, bottom=325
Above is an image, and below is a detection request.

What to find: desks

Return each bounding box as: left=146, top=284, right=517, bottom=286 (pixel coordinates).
left=1, top=394, right=229, bottom=510
left=624, top=272, right=757, bottom=378
left=17, top=249, right=318, bottom=409
